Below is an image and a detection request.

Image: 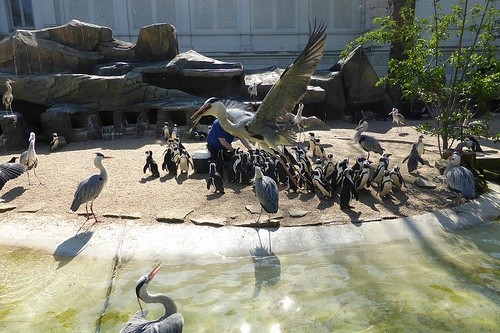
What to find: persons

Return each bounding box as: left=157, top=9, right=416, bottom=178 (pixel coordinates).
left=205, top=112, right=253, bottom=178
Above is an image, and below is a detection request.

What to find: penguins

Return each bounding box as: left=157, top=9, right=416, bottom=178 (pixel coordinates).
left=403, top=134, right=427, bottom=175
left=50, top=132, right=59, bottom=152
left=143, top=121, right=202, bottom=180
left=206, top=132, right=408, bottom=211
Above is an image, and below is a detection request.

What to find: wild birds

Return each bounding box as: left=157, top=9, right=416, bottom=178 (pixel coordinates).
left=440, top=134, right=484, bottom=217
left=0, top=79, right=42, bottom=193
left=354, top=108, right=406, bottom=163
left=251, top=162, right=280, bottom=224
left=70, top=153, right=115, bottom=227
left=190, top=16, right=329, bottom=190
left=119, top=260, right=185, bottom=333
